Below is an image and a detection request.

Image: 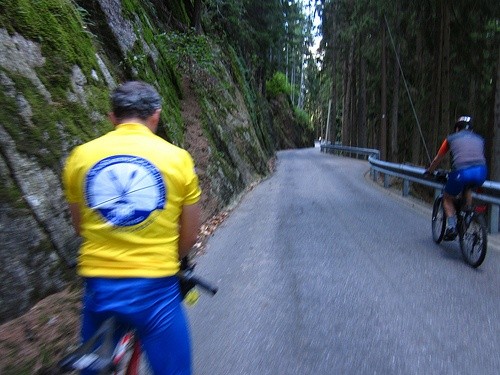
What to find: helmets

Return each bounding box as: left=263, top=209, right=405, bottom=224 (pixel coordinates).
left=456, top=116, right=474, bottom=128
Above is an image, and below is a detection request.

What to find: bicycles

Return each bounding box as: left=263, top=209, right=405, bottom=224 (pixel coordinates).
left=422, top=170, right=489, bottom=269
left=52, top=258, right=218, bottom=375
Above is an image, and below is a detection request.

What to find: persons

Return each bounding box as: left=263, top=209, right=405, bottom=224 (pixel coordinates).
left=425, top=115, right=487, bottom=242
left=60, top=83, right=201, bottom=375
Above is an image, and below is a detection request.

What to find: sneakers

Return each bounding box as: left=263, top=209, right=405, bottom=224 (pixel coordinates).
left=443, top=229, right=456, bottom=243
